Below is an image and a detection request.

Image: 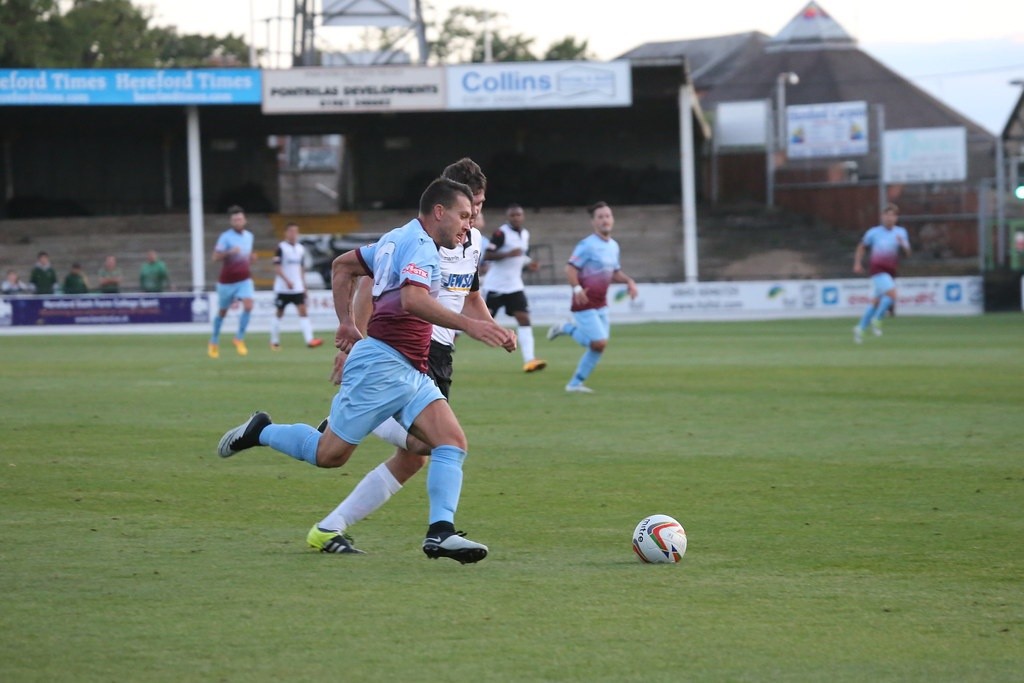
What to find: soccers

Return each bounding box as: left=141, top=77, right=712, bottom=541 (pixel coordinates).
left=632, top=512, right=688, bottom=565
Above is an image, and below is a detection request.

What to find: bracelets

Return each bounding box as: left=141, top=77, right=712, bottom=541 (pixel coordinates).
left=574, top=285, right=584, bottom=294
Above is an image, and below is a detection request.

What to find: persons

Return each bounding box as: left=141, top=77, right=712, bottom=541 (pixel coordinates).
left=270, top=222, right=325, bottom=348
left=304, top=158, right=516, bottom=555
left=1, top=271, right=28, bottom=294
left=851, top=200, right=911, bottom=345
left=63, top=261, right=91, bottom=293
left=483, top=204, right=547, bottom=372
left=546, top=201, right=640, bottom=392
left=207, top=206, right=255, bottom=357
left=140, top=248, right=172, bottom=292
left=97, top=255, right=124, bottom=294
left=29, top=251, right=59, bottom=294
left=218, top=173, right=512, bottom=566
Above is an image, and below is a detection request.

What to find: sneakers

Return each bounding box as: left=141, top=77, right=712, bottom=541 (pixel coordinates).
left=307, top=524, right=366, bottom=554
left=217, top=410, right=273, bottom=460
left=422, top=528, right=489, bottom=565
left=317, top=415, right=329, bottom=433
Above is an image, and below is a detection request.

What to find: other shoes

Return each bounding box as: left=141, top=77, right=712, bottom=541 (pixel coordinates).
left=565, top=383, right=594, bottom=393
left=852, top=325, right=864, bottom=345
left=546, top=320, right=569, bottom=341
left=232, top=338, right=247, bottom=356
left=271, top=343, right=281, bottom=352
left=307, top=338, right=323, bottom=348
left=523, top=360, right=546, bottom=374
left=871, top=317, right=883, bottom=336
left=207, top=343, right=218, bottom=357
